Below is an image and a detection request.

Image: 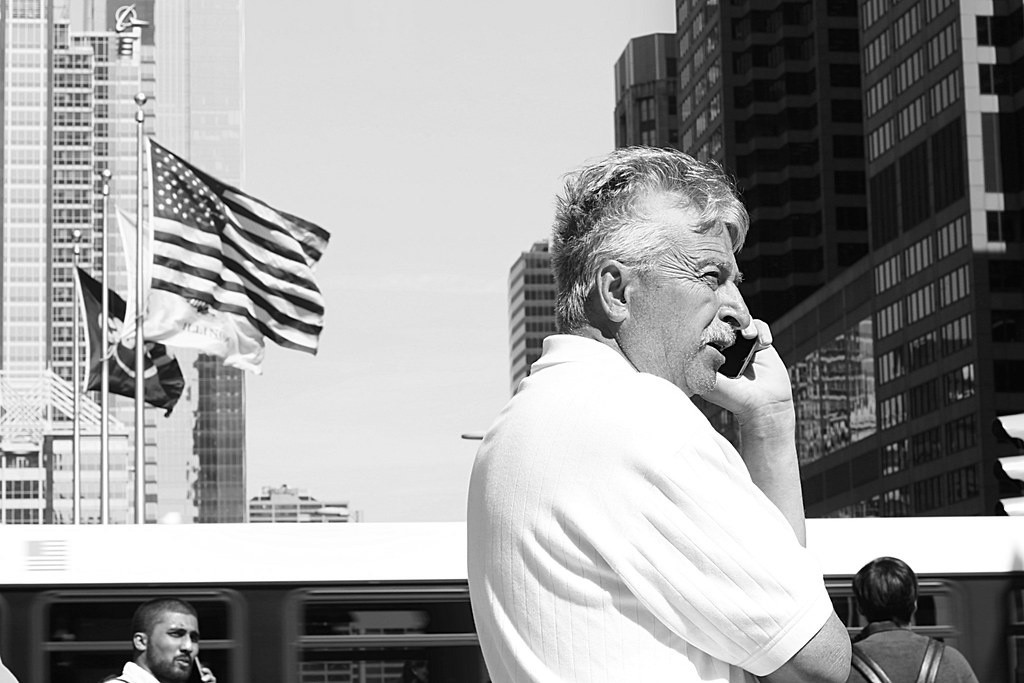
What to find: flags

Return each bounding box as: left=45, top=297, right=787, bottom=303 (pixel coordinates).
left=144, top=137, right=331, bottom=356
left=117, top=202, right=267, bottom=376
left=74, top=265, right=185, bottom=418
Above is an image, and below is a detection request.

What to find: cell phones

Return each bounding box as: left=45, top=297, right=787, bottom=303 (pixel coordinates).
left=186, top=657, right=205, bottom=682
left=717, top=330, right=758, bottom=379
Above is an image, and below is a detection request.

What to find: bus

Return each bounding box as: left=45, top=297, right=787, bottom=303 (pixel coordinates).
left=0, top=516, right=1023, bottom=683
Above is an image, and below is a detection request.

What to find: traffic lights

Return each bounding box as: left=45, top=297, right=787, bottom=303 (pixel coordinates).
left=996, top=411, right=1024, bottom=517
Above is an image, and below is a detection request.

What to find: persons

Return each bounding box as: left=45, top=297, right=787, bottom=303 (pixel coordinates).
left=467, top=146, right=852, bottom=683
left=847, top=555, right=981, bottom=683
left=99, top=596, right=219, bottom=683
